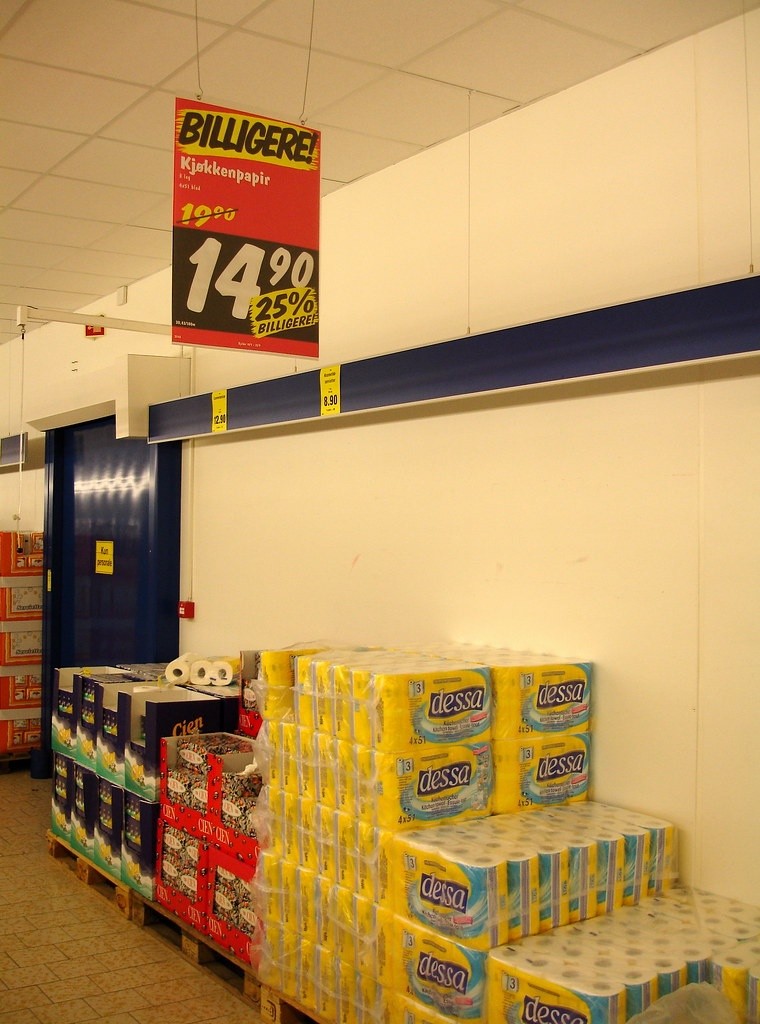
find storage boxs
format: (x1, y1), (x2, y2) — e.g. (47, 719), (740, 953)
(52, 662), (263, 970)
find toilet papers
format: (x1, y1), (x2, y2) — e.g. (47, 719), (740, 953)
(42, 636), (760, 1024)
